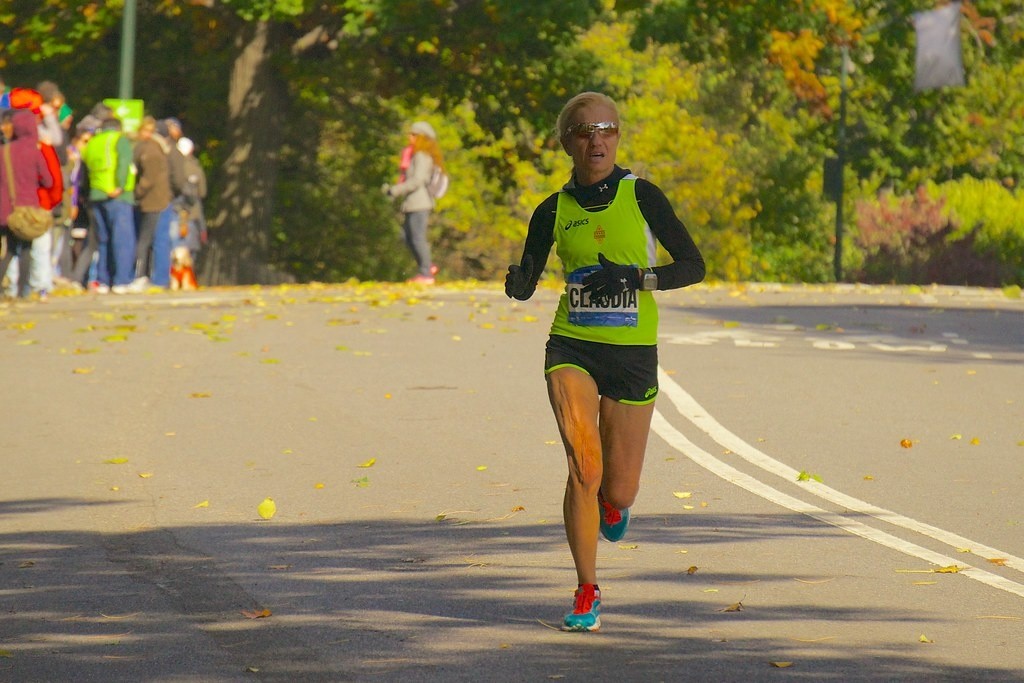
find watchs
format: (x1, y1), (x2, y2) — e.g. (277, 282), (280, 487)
(640, 267), (658, 291)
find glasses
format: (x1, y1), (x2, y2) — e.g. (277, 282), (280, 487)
(563, 122), (619, 137)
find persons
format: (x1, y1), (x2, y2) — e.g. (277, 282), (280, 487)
(382, 122), (449, 286)
(0, 77), (208, 308)
(505, 92), (707, 632)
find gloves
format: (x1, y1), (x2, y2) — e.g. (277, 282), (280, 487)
(580, 252), (641, 300)
(504, 254), (538, 301)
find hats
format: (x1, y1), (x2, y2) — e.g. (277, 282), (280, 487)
(406, 121), (437, 140)
(8, 87), (44, 110)
(176, 137), (193, 156)
(155, 119), (169, 137)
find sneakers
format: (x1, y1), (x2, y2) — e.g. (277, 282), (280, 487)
(597, 486), (630, 544)
(561, 584), (602, 632)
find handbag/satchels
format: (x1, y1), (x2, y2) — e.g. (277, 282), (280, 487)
(6, 205), (53, 241)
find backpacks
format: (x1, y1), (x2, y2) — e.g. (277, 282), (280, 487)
(425, 164), (449, 199)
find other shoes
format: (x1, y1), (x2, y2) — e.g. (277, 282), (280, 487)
(97, 286), (109, 294)
(39, 297), (48, 303)
(429, 263), (439, 276)
(111, 285), (126, 295)
(406, 273), (435, 287)
(3, 289), (18, 297)
